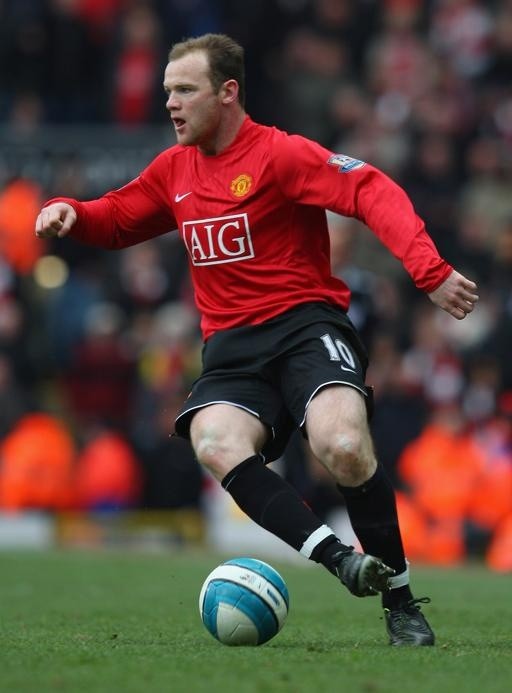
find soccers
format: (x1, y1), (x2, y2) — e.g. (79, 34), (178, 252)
(200, 558), (289, 645)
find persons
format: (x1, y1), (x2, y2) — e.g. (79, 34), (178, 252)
(35, 33), (478, 645)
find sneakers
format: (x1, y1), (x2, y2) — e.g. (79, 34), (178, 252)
(330, 546), (395, 597)
(384, 595), (435, 645)
